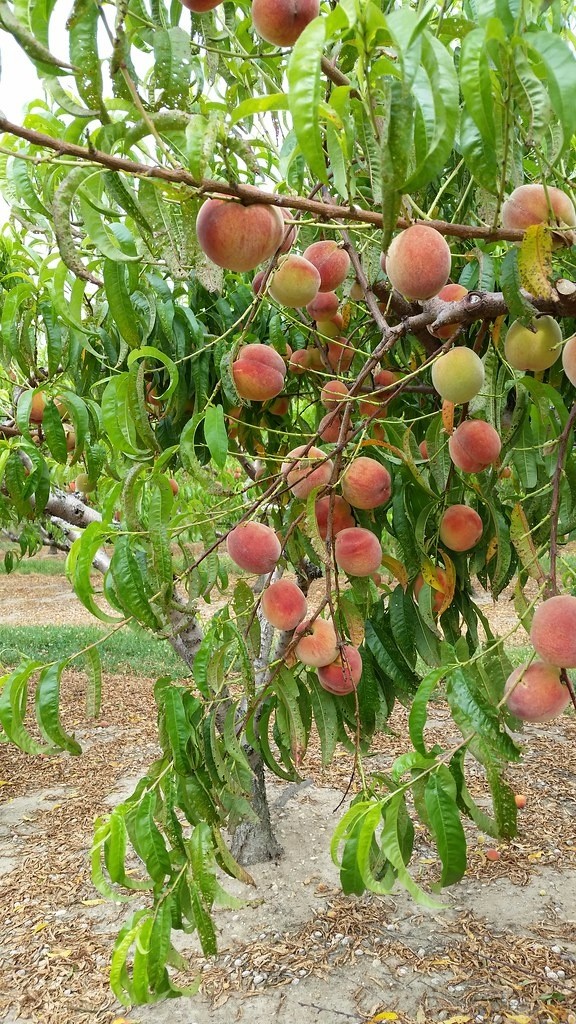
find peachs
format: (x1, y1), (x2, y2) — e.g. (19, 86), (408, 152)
(8, 1), (576, 862)
